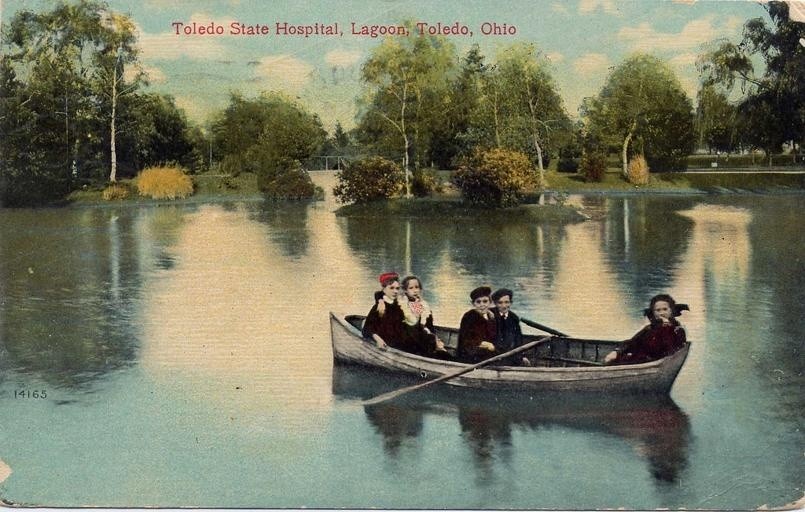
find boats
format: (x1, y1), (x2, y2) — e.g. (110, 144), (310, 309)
(329, 310), (693, 394)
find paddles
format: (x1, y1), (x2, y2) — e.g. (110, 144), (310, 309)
(359, 338), (551, 406)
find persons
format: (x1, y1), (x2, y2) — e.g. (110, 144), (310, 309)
(491, 290), (524, 365)
(362, 273), (409, 351)
(457, 288), (496, 364)
(401, 277), (452, 360)
(604, 294), (686, 368)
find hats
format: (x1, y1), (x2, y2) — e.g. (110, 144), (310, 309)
(379, 272), (399, 283)
(470, 287), (491, 299)
(492, 288), (512, 303)
